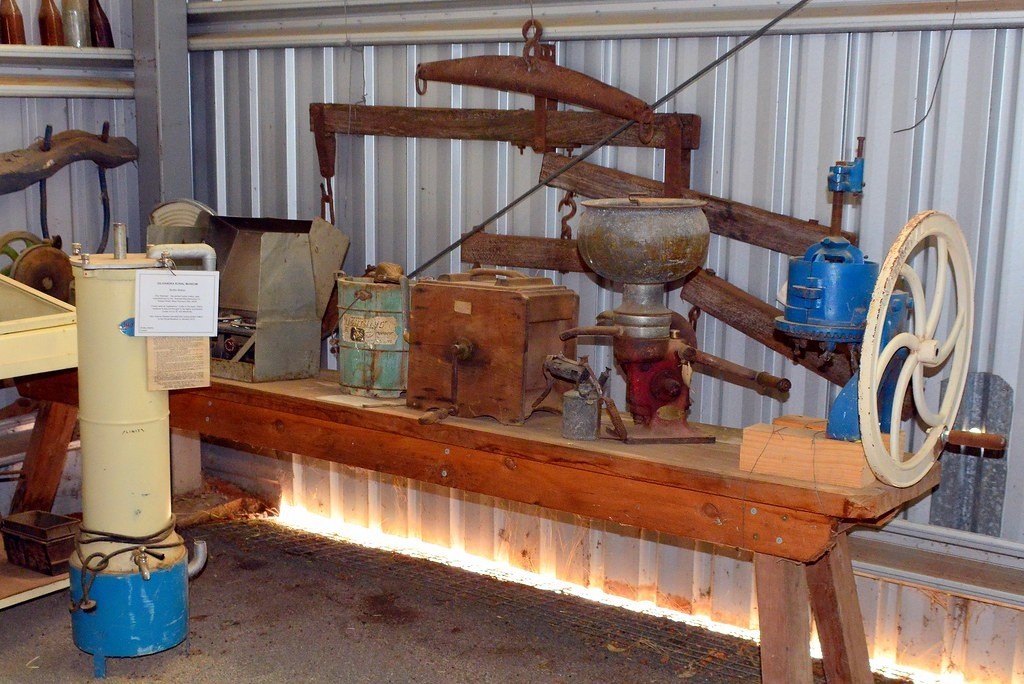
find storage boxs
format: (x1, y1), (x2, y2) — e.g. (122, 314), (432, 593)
(2, 510), (80, 575)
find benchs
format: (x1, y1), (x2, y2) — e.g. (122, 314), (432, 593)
(8, 368), (940, 684)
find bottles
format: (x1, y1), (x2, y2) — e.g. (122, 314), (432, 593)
(38, 0), (64, 46)
(88, 0), (114, 48)
(0, 0), (27, 45)
(59, 0), (92, 48)
